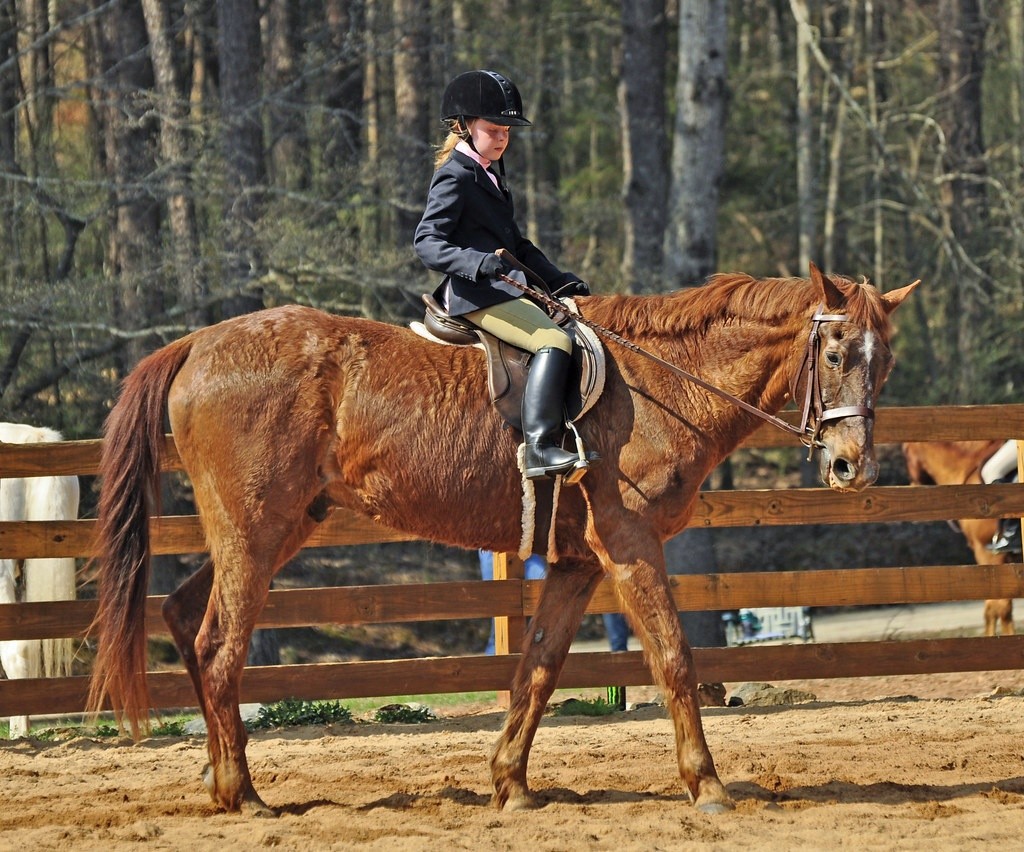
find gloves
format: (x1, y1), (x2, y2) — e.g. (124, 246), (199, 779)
(479, 253), (504, 279)
(573, 283), (591, 295)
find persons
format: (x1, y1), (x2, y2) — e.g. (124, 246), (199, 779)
(478, 549), (547, 653)
(414, 70), (605, 479)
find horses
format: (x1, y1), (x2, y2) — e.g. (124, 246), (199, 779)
(85, 261), (922, 812)
(896, 423), (1024, 642)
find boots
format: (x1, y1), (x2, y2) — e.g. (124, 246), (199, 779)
(983, 517), (1022, 554)
(521, 347), (603, 479)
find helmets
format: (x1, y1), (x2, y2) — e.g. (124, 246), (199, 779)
(441, 69), (532, 126)
